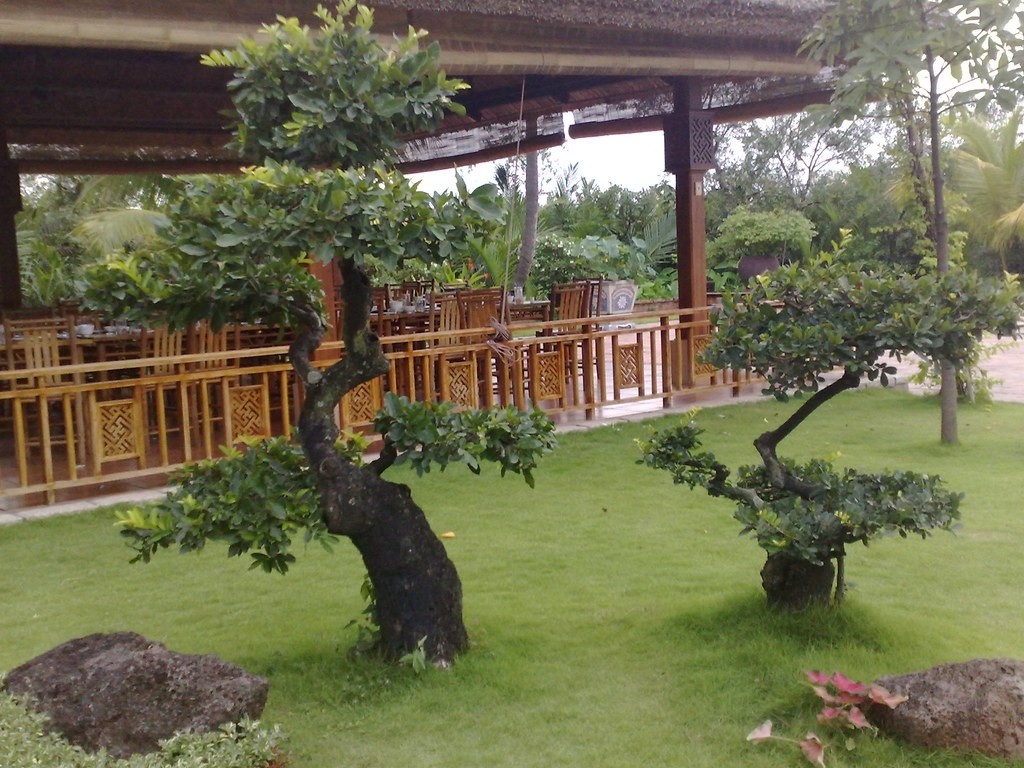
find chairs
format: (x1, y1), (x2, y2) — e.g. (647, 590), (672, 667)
(536, 276), (603, 384)
(1, 279), (536, 489)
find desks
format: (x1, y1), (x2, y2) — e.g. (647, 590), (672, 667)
(0, 298), (551, 399)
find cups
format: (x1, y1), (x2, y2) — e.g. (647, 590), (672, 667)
(80, 324), (94, 337)
(514, 286), (523, 305)
(389, 301), (403, 311)
(413, 297), (427, 312)
(507, 296), (514, 303)
(529, 296), (535, 304)
(405, 305), (416, 314)
